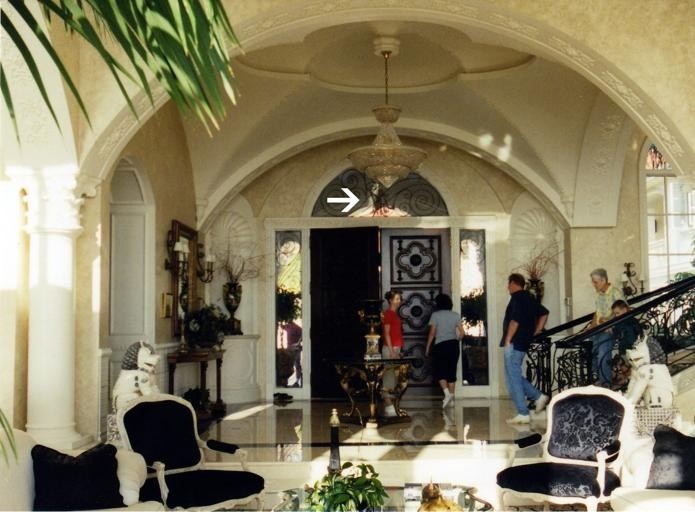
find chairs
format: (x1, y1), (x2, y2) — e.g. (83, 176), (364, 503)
(495, 385), (634, 512)
(115, 393), (266, 511)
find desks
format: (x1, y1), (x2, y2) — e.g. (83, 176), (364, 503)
(168, 349), (225, 424)
(332, 357), (411, 428)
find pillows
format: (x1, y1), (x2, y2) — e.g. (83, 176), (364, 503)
(31, 443), (128, 512)
(645, 423), (695, 491)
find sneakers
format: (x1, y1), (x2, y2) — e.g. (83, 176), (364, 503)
(532, 393), (549, 414)
(441, 393), (452, 409)
(448, 400), (455, 408)
(505, 413), (531, 426)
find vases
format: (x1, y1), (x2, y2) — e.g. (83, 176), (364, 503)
(221, 280), (243, 335)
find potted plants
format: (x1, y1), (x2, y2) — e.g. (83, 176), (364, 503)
(513, 241), (562, 303)
(181, 388), (212, 435)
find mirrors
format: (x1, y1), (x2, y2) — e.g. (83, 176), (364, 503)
(171, 218), (198, 338)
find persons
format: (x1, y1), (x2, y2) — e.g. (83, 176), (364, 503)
(281, 318), (302, 388)
(588, 268), (628, 386)
(602, 300), (642, 382)
(425, 292), (465, 408)
(381, 290), (403, 416)
(499, 272), (550, 425)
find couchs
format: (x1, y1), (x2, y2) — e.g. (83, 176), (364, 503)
(609, 424), (695, 512)
(0, 427), (165, 512)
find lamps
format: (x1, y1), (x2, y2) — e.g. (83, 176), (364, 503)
(165, 241), (190, 278)
(199, 254), (216, 283)
(620, 262), (645, 300)
(346, 49), (429, 190)
